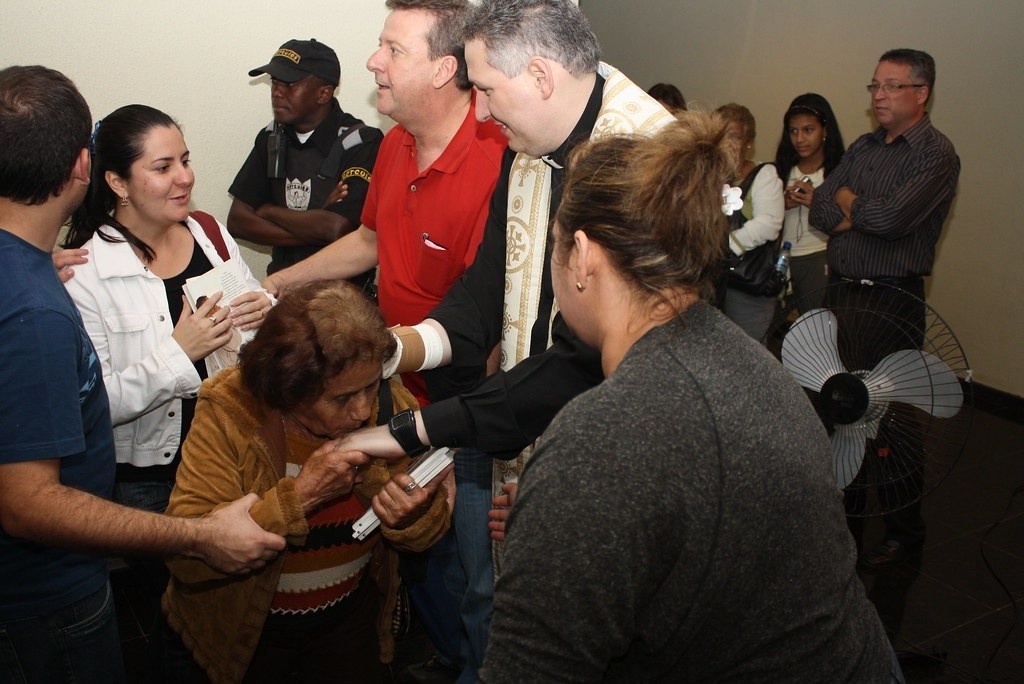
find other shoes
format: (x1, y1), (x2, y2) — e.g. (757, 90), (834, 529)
(404, 656), (462, 684)
(858, 540), (925, 566)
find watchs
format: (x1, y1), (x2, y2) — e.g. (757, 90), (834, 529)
(389, 408), (431, 457)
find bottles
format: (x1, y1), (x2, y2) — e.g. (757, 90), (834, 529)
(774, 241), (792, 276)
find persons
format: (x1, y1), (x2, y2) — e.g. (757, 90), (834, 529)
(806, 48), (962, 473)
(709, 103), (785, 354)
(334, 0), (686, 589)
(65, 104), (283, 684)
(257, 1), (508, 411)
(227, 39), (390, 293)
(161, 274), (454, 684)
(773, 93), (845, 313)
(481, 100), (904, 684)
(0, 64), (287, 684)
(647, 83), (688, 118)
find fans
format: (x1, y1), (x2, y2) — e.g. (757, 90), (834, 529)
(756, 276), (974, 584)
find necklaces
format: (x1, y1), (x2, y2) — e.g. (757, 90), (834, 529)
(280, 413), (302, 469)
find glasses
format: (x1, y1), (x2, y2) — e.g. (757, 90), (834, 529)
(867, 84), (927, 94)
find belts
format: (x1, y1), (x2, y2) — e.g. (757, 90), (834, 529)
(872, 275), (924, 288)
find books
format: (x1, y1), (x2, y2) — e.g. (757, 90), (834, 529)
(349, 446), (456, 542)
(182, 258), (261, 378)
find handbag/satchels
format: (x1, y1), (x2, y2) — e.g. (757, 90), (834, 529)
(713, 162), (789, 296)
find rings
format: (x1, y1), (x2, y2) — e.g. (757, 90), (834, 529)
(403, 481), (418, 492)
(210, 315), (216, 322)
(353, 464), (360, 470)
(258, 308), (264, 317)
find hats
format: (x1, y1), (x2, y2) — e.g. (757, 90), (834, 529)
(249, 39), (340, 85)
